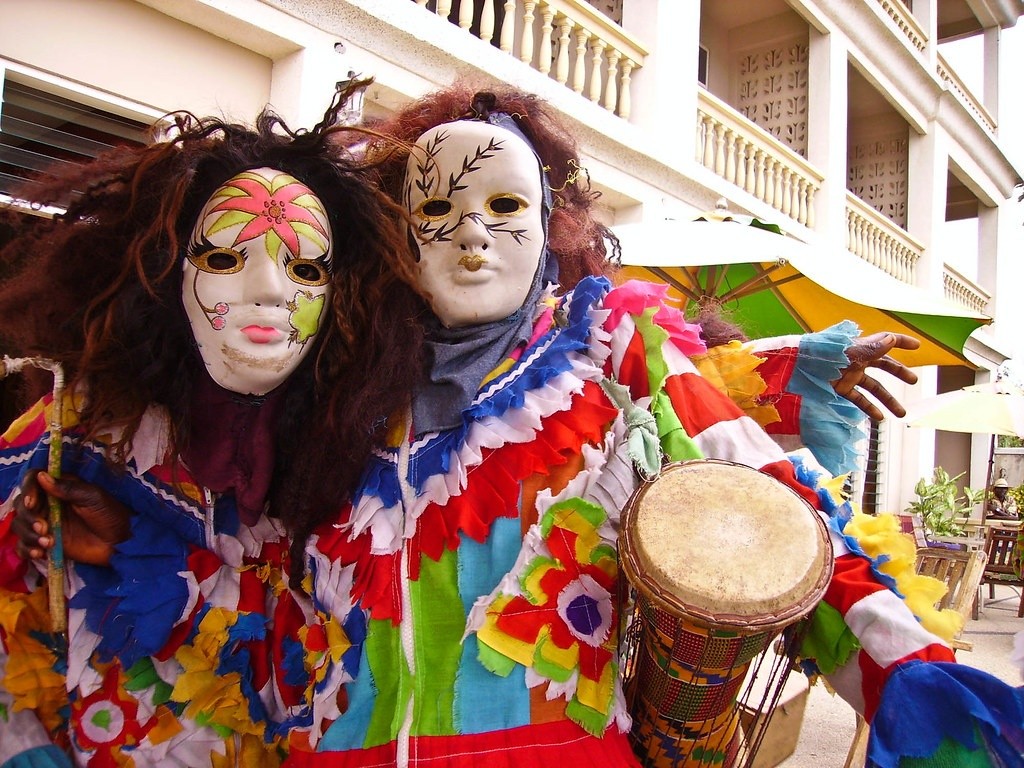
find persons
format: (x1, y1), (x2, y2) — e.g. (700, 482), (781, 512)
(0, 85), (1024, 768)
(984, 478), (1018, 553)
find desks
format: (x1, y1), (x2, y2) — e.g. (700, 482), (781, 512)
(929, 536), (1013, 613)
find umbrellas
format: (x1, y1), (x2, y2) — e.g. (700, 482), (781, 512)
(605, 198), (995, 371)
(898, 380), (1024, 539)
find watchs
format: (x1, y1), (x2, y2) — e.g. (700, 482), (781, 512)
(994, 508), (1000, 513)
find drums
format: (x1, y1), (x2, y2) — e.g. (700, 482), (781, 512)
(617, 455), (836, 768)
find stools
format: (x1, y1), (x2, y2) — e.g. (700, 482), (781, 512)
(737, 660), (811, 768)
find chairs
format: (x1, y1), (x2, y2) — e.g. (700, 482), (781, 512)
(844, 515), (1024, 768)
